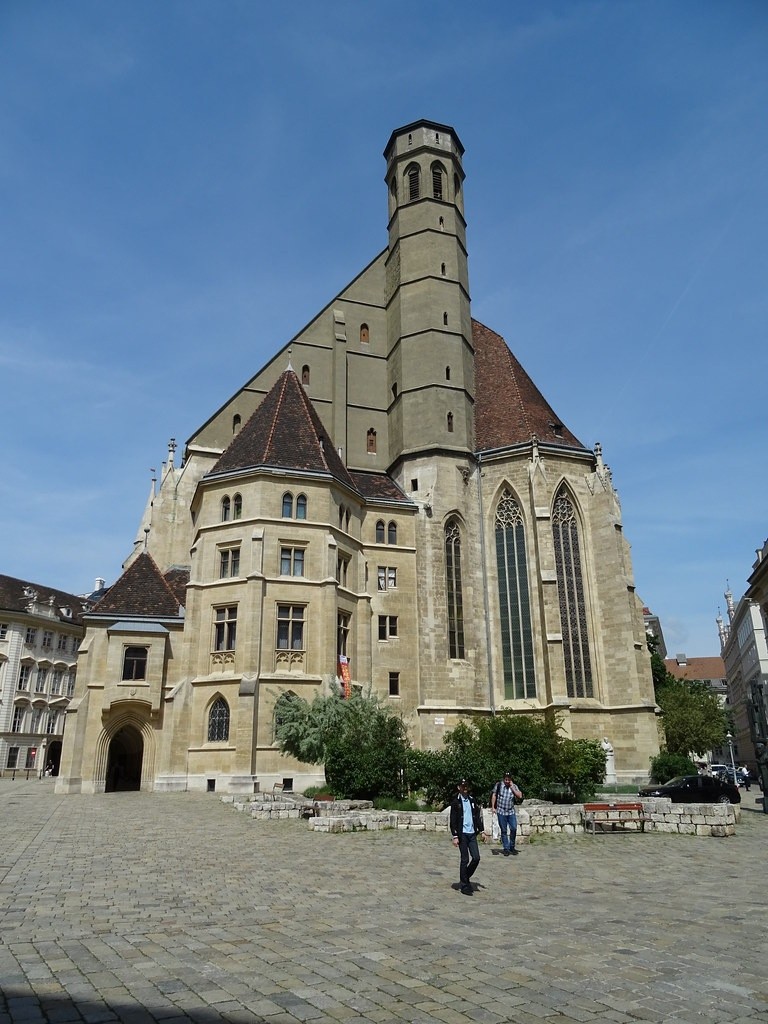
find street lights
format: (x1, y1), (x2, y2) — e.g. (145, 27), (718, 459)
(727, 730), (741, 787)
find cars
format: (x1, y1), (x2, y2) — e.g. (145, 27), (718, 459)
(698, 762), (747, 787)
(637, 774), (742, 805)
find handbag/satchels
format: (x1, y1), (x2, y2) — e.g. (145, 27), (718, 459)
(494, 782), (501, 809)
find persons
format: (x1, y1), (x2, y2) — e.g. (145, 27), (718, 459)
(491, 772), (523, 856)
(742, 763), (751, 792)
(45, 759), (54, 778)
(450, 778), (486, 896)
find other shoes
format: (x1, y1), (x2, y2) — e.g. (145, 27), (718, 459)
(504, 849), (509, 856)
(461, 882), (473, 896)
(510, 848), (517, 855)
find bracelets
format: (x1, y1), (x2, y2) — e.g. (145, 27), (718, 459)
(452, 836), (458, 839)
(480, 831), (485, 835)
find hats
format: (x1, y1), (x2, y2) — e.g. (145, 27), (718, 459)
(503, 770), (511, 777)
(458, 778), (468, 786)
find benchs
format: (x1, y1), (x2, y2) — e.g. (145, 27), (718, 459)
(301, 794), (336, 818)
(263, 783), (285, 802)
(584, 802), (652, 836)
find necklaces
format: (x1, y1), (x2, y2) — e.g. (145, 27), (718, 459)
(464, 800), (468, 802)
